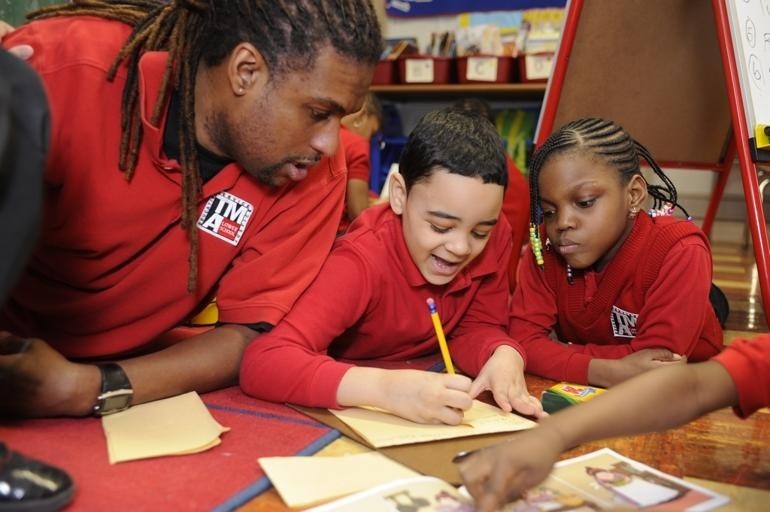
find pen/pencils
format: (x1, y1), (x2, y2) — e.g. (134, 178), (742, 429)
(429, 32), (457, 60)
(426, 298), (455, 376)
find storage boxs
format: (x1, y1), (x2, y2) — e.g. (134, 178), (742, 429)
(370, 59), (398, 85)
(397, 55), (457, 84)
(456, 55), (518, 83)
(518, 51), (555, 83)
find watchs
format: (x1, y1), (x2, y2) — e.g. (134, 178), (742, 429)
(89, 361), (134, 418)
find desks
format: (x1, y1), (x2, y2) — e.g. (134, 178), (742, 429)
(0, 372), (770, 512)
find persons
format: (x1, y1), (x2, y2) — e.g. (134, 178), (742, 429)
(336, 90), (383, 237)
(0, 0), (383, 421)
(238, 108), (550, 426)
(454, 330), (768, 512)
(506, 115), (730, 389)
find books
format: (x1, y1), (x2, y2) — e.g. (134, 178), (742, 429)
(304, 446), (730, 511)
